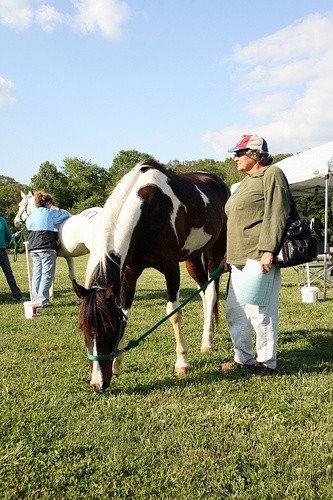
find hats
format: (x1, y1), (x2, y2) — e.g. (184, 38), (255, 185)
(227, 134), (269, 154)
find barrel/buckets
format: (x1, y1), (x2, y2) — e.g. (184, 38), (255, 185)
(24, 301), (42, 319)
(301, 287), (318, 303)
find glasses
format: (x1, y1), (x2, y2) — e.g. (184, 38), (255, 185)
(233, 151), (249, 158)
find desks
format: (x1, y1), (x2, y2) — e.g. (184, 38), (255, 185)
(296, 263), (333, 288)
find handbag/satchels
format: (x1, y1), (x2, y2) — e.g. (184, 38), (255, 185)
(273, 216), (323, 269)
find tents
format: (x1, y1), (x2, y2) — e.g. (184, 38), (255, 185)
(231, 139), (333, 301)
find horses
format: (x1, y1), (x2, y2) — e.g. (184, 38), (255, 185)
(12, 189), (105, 288)
(71, 156), (231, 395)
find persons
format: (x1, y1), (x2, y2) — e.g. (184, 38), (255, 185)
(0, 204), (28, 300)
(28, 192), (70, 308)
(221, 134), (291, 375)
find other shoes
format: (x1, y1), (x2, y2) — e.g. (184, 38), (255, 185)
(13, 296), (30, 301)
(246, 364), (276, 380)
(218, 359), (255, 372)
